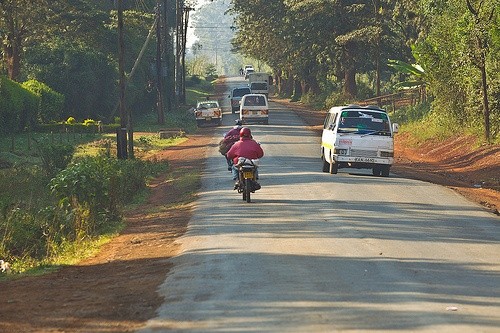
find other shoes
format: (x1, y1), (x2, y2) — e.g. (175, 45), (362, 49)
(233, 180), (239, 189)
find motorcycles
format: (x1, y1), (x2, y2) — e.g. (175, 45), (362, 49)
(225, 143), (260, 203)
(222, 132), (238, 174)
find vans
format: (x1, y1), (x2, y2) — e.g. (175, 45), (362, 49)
(228, 87), (252, 114)
(245, 68), (255, 80)
(320, 103), (394, 177)
(239, 94), (269, 125)
(243, 65), (254, 75)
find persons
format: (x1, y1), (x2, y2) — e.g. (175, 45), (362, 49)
(338, 117), (350, 128)
(227, 128), (264, 190)
(240, 68), (243, 75)
(223, 119), (252, 171)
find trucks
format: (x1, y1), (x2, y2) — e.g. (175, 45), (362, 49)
(248, 72), (273, 102)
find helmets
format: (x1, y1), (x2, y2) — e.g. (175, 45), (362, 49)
(234, 118), (242, 125)
(239, 127), (251, 138)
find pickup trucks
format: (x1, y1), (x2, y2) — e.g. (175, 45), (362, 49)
(194, 101), (223, 128)
